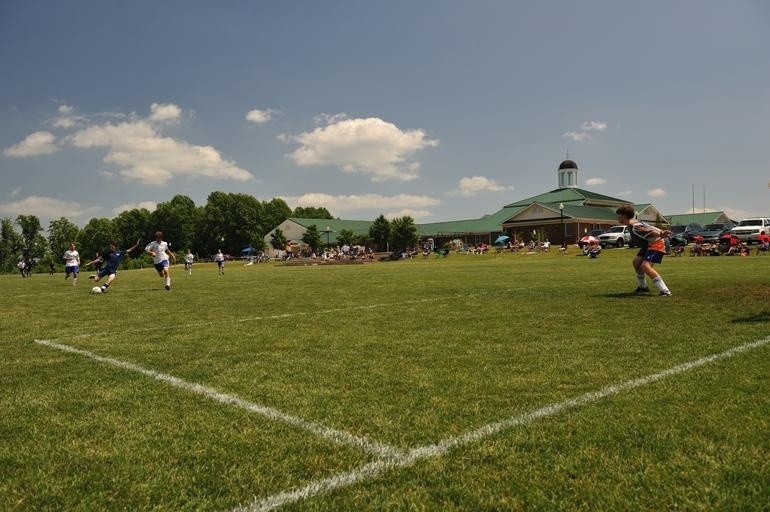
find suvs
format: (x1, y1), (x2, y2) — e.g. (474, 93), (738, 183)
(596, 224), (632, 247)
(731, 216), (770, 244)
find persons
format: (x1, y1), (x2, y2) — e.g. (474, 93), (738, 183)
(665, 231), (769, 256)
(470, 243), (488, 255)
(250, 249), (311, 263)
(18, 259), (32, 278)
(63, 243), (80, 286)
(311, 243), (375, 263)
(184, 249), (194, 276)
(49, 260), (55, 275)
(615, 206), (672, 297)
(145, 232), (176, 290)
(402, 244), (430, 259)
(215, 250), (224, 275)
(85, 238), (140, 292)
(583, 242), (602, 258)
(499, 239), (567, 253)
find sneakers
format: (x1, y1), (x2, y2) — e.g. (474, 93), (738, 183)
(165, 286), (170, 290)
(635, 286), (672, 296)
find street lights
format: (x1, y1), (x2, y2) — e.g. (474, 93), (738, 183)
(327, 226), (330, 251)
(558, 203), (565, 247)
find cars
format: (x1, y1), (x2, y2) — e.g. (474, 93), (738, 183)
(694, 222), (732, 245)
(578, 230), (604, 249)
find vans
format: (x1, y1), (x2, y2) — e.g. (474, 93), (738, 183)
(667, 223), (702, 246)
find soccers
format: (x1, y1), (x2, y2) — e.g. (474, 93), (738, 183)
(92, 287), (102, 293)
